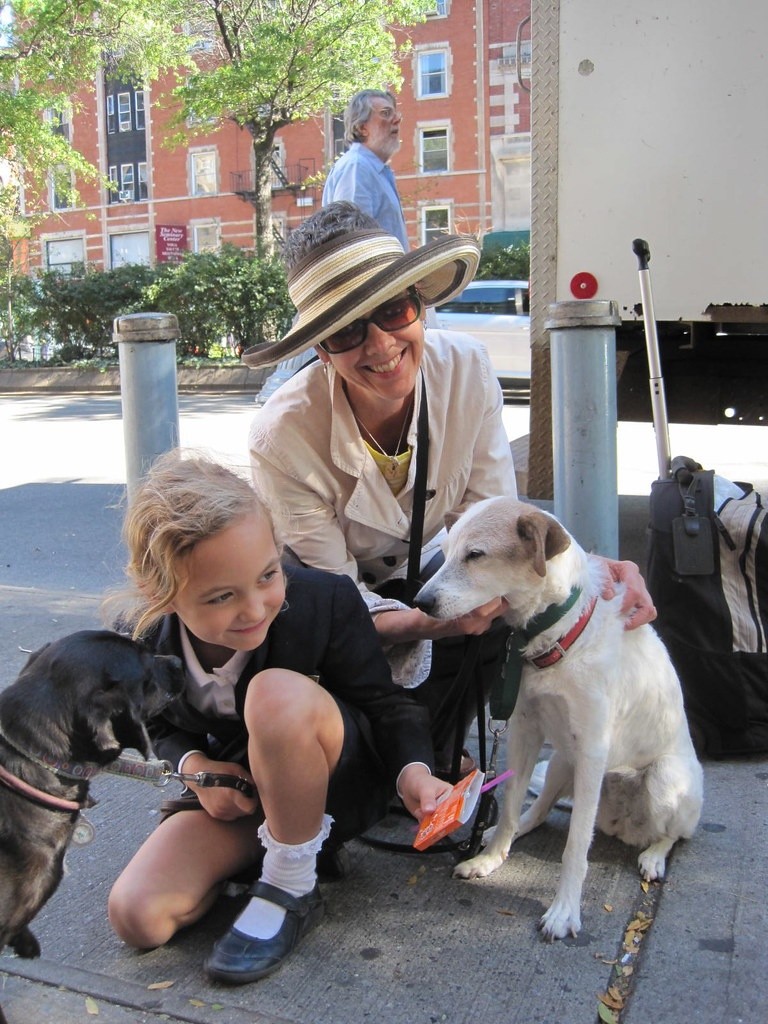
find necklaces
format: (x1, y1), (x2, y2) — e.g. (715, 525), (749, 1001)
(355, 395), (413, 471)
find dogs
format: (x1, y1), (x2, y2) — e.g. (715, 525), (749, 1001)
(413, 495), (706, 943)
(0, 629), (185, 961)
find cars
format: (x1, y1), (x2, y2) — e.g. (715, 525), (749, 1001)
(424, 281), (531, 400)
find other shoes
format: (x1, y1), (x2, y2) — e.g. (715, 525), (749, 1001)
(435, 749), (476, 778)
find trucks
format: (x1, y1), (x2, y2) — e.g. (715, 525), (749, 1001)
(516, 1), (768, 499)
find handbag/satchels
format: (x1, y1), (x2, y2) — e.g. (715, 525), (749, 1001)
(369, 578), (422, 608)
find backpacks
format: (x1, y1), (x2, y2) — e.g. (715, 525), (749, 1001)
(644, 455), (768, 758)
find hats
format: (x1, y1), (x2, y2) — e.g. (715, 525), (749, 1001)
(241, 228), (482, 368)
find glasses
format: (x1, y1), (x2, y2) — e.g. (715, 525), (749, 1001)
(319, 287), (421, 354)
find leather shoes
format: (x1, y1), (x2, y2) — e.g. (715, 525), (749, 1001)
(203, 882), (325, 982)
(315, 834), (351, 881)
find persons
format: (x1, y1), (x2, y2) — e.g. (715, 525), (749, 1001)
(107, 453), (455, 985)
(244, 201), (657, 780)
(320, 90), (411, 254)
